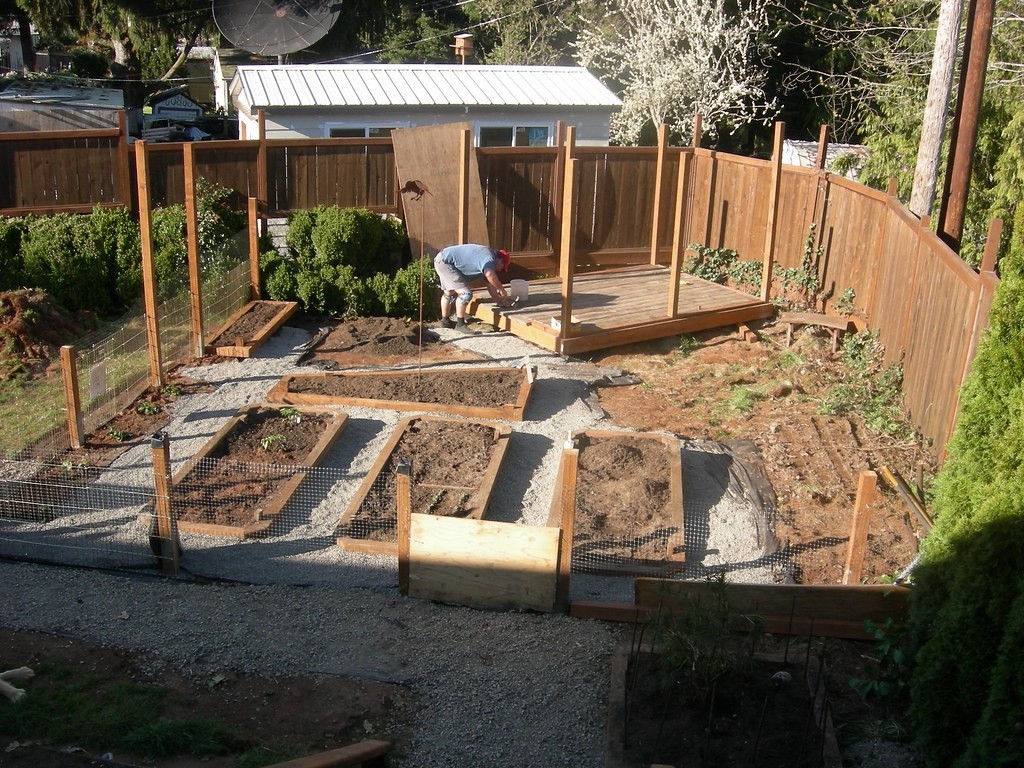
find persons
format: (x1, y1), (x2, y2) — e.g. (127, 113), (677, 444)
(434, 245), (510, 334)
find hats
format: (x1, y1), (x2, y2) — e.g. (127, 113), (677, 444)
(494, 249), (510, 272)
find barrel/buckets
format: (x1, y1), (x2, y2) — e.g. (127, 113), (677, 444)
(510, 278), (529, 302)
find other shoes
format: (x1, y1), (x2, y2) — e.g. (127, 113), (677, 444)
(441, 321), (457, 328)
(455, 324), (474, 335)
